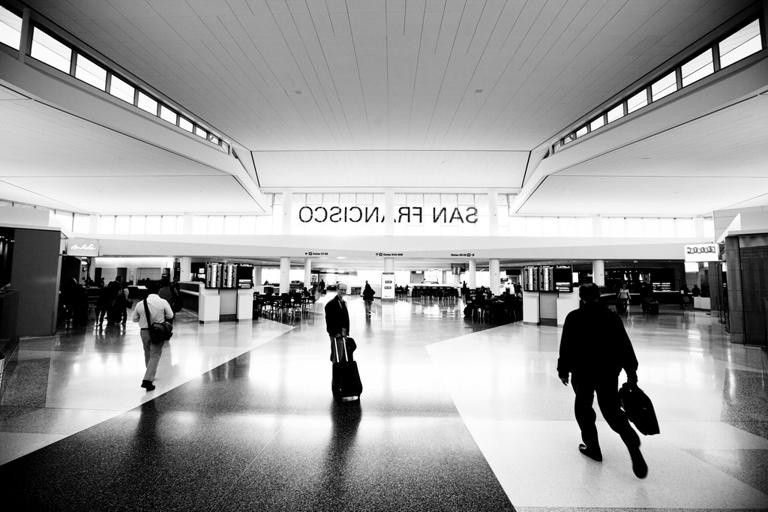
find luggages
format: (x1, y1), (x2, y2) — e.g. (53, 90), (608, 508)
(333, 361), (362, 398)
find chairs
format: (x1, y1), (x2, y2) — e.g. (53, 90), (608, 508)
(461, 285), (523, 329)
(411, 286), (460, 304)
(257, 288), (316, 323)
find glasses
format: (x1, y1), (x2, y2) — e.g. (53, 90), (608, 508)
(337, 287), (348, 292)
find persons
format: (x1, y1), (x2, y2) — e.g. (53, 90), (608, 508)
(132, 283), (173, 389)
(640, 281), (654, 315)
(362, 281), (376, 306)
(325, 283), (349, 362)
(299, 279), (326, 303)
(557, 282), (647, 478)
(68, 274), (183, 332)
(692, 284), (700, 296)
(615, 282), (631, 314)
(679, 284), (691, 310)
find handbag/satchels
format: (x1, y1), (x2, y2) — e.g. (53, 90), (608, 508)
(147, 319), (174, 342)
(620, 386), (663, 438)
(330, 337), (357, 361)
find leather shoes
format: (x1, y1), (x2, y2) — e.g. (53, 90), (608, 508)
(577, 442), (603, 463)
(626, 450), (649, 481)
(139, 381), (158, 391)
